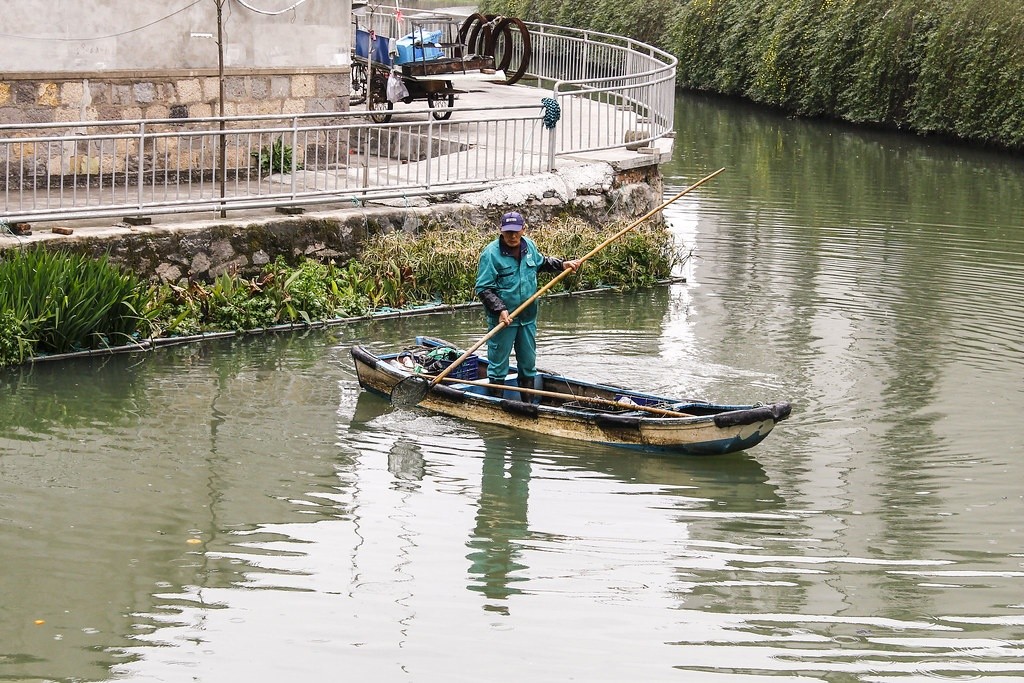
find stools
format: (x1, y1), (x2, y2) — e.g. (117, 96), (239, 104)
(394, 29), (445, 66)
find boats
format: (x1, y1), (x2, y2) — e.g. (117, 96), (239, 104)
(351, 333), (792, 458)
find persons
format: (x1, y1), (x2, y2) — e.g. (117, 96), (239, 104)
(474, 212), (580, 403)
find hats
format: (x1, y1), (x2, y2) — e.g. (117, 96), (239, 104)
(500, 211), (524, 232)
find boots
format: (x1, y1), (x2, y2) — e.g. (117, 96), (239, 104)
(516, 374), (534, 403)
(490, 378), (504, 398)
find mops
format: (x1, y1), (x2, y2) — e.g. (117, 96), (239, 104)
(510, 97), (561, 177)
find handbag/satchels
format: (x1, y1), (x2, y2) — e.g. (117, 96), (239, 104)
(386, 69), (409, 102)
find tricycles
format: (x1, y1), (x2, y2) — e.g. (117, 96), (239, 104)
(349, 38), (457, 124)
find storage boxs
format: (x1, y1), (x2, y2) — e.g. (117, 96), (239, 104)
(437, 355), (479, 384)
